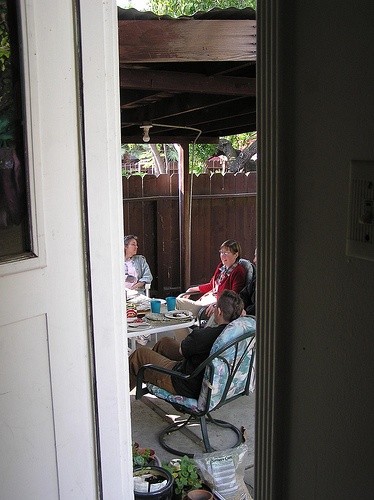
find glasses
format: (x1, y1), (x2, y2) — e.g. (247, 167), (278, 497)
(219, 251), (235, 255)
(213, 304), (218, 308)
(128, 244), (139, 246)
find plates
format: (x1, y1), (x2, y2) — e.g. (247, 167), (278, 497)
(164, 309), (193, 319)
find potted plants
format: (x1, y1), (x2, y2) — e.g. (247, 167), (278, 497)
(161, 456), (203, 500)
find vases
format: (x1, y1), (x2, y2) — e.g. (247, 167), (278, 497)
(133, 466), (175, 500)
(133, 455), (162, 469)
(187, 489), (214, 500)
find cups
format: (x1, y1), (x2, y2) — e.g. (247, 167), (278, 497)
(150, 301), (161, 314)
(166, 297), (177, 311)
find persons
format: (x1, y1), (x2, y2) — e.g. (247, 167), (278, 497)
(253, 248), (257, 265)
(124, 234), (153, 311)
(128, 288), (244, 398)
(175, 239), (248, 318)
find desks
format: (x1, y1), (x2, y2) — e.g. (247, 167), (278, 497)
(127, 305), (195, 354)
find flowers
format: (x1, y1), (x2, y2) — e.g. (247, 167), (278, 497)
(132, 441), (156, 467)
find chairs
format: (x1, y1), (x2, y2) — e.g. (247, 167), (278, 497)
(176, 259), (257, 319)
(136, 315), (255, 458)
